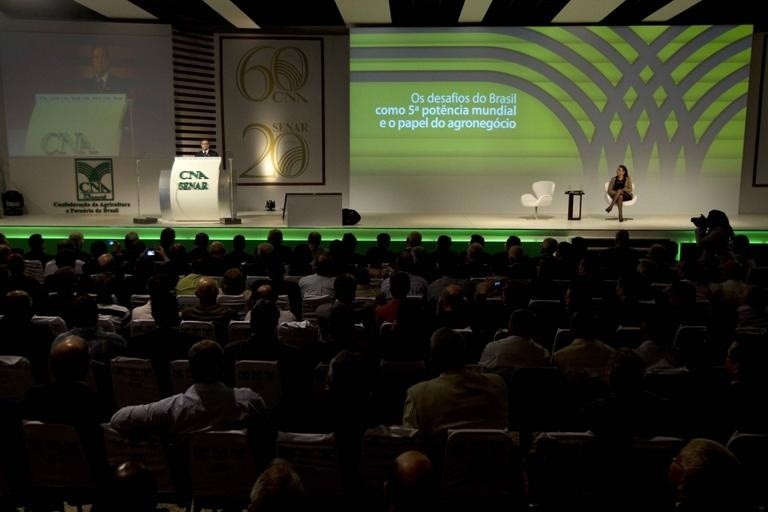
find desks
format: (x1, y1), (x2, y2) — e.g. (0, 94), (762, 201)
(564, 189), (585, 219)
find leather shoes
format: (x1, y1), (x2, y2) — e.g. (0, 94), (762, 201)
(605, 208), (610, 213)
(620, 218), (623, 221)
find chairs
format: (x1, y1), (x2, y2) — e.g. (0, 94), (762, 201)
(605, 180), (636, 219)
(521, 180), (556, 219)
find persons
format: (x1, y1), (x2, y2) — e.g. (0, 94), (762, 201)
(79, 45), (124, 93)
(694, 209), (738, 264)
(1, 226), (768, 509)
(606, 164), (633, 224)
(193, 139), (219, 157)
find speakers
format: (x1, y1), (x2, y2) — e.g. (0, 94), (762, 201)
(342, 208), (362, 226)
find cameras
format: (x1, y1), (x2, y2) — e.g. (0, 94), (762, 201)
(493, 280), (502, 289)
(692, 214), (708, 227)
(145, 249), (158, 257)
(107, 240), (115, 247)
